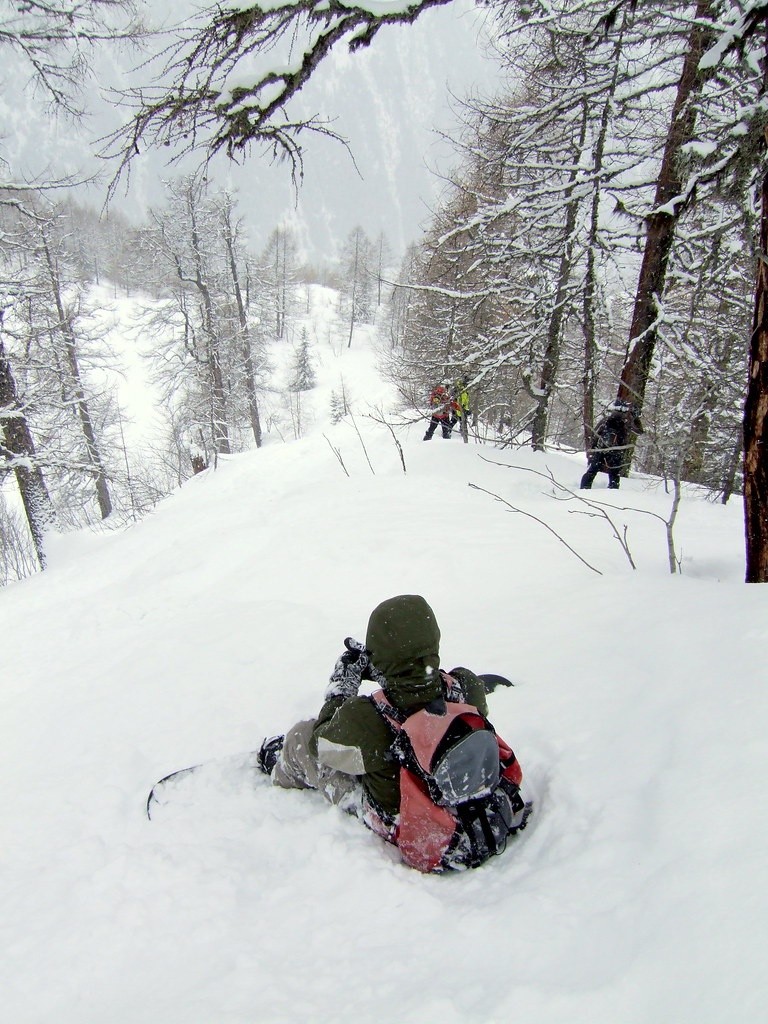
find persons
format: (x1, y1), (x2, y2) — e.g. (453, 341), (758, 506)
(422, 377), (471, 440)
(580, 401), (644, 490)
(258, 595), (488, 845)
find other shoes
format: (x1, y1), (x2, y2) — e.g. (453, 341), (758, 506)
(260, 734), (285, 773)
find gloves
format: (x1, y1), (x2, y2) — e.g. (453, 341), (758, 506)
(324, 650), (370, 697)
(630, 405), (642, 417)
(343, 637), (386, 685)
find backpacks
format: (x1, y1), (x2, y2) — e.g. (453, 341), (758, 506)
(595, 417), (627, 455)
(367, 671), (525, 872)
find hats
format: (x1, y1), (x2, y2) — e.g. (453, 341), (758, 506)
(614, 401), (629, 414)
(442, 379), (451, 385)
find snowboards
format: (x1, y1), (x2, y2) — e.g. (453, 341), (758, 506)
(146, 749), (259, 820)
